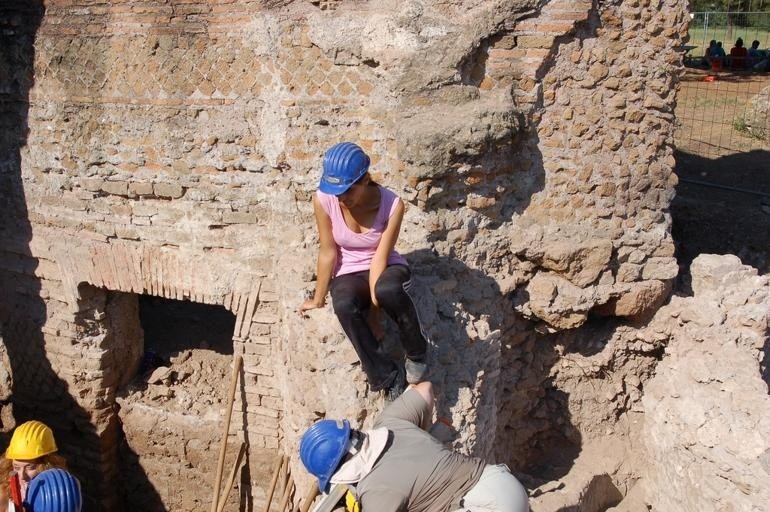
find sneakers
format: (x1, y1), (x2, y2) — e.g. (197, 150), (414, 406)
(405, 359), (426, 384)
(384, 368), (405, 400)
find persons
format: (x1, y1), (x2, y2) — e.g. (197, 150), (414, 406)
(700, 37), (770, 72)
(299, 378), (533, 511)
(4, 419), (59, 511)
(298, 140), (428, 403)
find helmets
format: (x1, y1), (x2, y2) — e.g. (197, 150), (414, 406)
(320, 142), (370, 195)
(299, 419), (350, 492)
(6, 420), (57, 460)
(26, 468), (82, 512)
